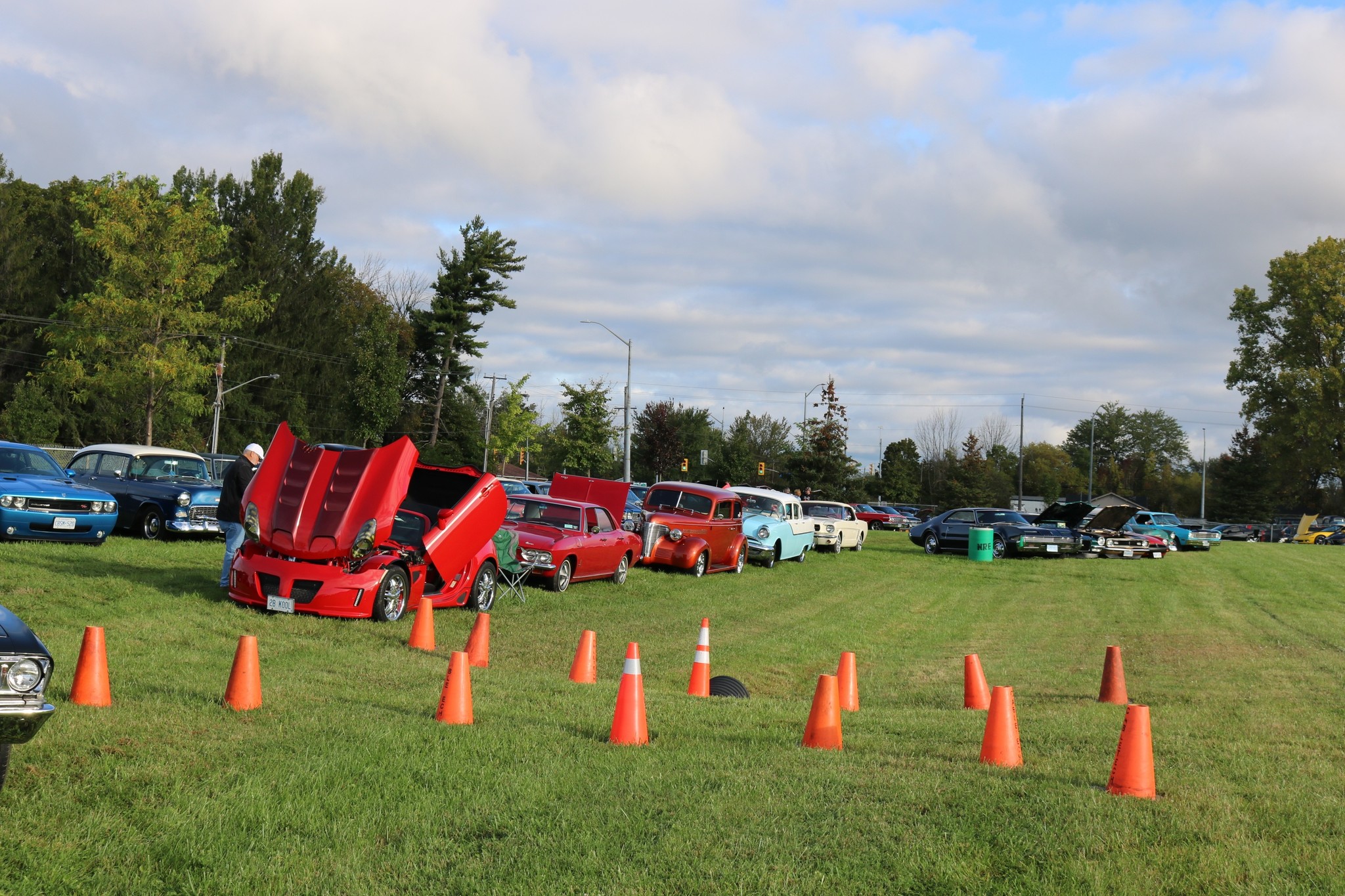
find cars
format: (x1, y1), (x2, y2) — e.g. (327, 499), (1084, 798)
(1211, 513), (1345, 546)
(0, 605), (56, 791)
(63, 443), (261, 539)
(843, 504), (934, 531)
(909, 500), (1221, 559)
(0, 440), (118, 546)
(492, 472), (651, 592)
(635, 481), (868, 580)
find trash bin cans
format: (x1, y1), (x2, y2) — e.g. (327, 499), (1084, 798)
(968, 527), (994, 562)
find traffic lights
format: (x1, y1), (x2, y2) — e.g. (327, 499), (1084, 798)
(520, 447), (524, 465)
(758, 462), (765, 476)
(681, 459), (688, 471)
(869, 464), (875, 476)
(493, 446), (498, 461)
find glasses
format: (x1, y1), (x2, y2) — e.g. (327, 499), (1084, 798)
(258, 455), (261, 461)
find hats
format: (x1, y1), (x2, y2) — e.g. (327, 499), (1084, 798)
(805, 487), (811, 491)
(245, 443), (264, 460)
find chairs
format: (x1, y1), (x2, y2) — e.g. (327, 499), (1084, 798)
(492, 530), (534, 603)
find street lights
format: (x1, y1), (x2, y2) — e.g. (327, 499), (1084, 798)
(1200, 428), (1206, 519)
(803, 384), (826, 445)
(844, 418), (852, 457)
(212, 374), (280, 454)
(580, 321), (632, 483)
(1088, 404), (1106, 505)
(690, 406), (725, 442)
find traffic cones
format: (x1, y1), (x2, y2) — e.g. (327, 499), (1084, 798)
(964, 653), (1155, 804)
(409, 597), (710, 745)
(801, 651), (860, 750)
(69, 626), (262, 707)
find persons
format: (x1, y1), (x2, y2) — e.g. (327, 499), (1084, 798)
(783, 486), (815, 501)
(216, 442), (264, 591)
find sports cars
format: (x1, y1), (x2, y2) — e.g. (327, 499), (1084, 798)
(228, 421), (508, 622)
